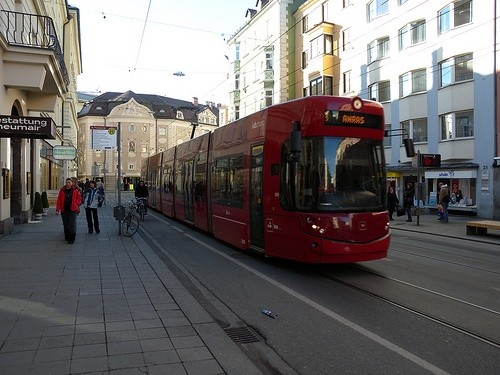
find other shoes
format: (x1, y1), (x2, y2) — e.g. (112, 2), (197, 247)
(389, 217), (394, 221)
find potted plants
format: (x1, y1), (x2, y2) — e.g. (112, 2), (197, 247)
(34, 192), (43, 220)
(41, 192), (49, 215)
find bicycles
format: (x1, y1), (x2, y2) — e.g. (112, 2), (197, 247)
(136, 196), (148, 221)
(122, 197), (143, 237)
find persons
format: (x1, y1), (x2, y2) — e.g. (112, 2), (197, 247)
(450, 188), (458, 204)
(81, 181), (104, 233)
(456, 190), (463, 203)
(439, 180), (450, 224)
(437, 183), (444, 220)
(388, 186), (400, 221)
(135, 181), (149, 215)
(69, 178), (105, 208)
(55, 178), (81, 245)
(404, 183), (414, 222)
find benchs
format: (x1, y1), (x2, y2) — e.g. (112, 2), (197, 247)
(466, 220), (500, 237)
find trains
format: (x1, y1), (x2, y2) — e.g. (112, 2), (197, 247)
(142, 94), (415, 267)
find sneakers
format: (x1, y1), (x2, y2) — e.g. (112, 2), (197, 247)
(406, 218), (412, 222)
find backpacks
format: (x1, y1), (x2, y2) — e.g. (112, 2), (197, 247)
(442, 193), (451, 204)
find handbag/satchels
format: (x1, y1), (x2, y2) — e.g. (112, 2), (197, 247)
(396, 204), (405, 216)
